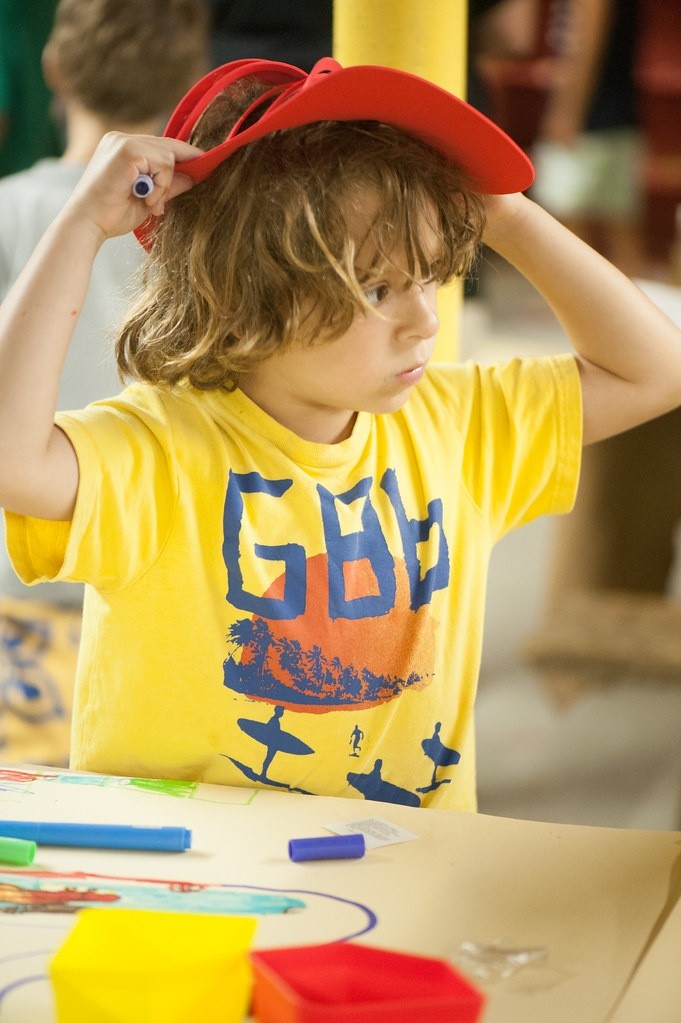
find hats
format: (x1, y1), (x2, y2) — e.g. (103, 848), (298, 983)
(131, 56), (536, 256)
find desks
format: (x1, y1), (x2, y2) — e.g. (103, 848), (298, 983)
(0, 761), (681, 1023)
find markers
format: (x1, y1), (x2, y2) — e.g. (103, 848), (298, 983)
(0, 820), (192, 867)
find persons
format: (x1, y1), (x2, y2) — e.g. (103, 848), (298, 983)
(0, 1), (208, 608)
(1, 56), (681, 813)
